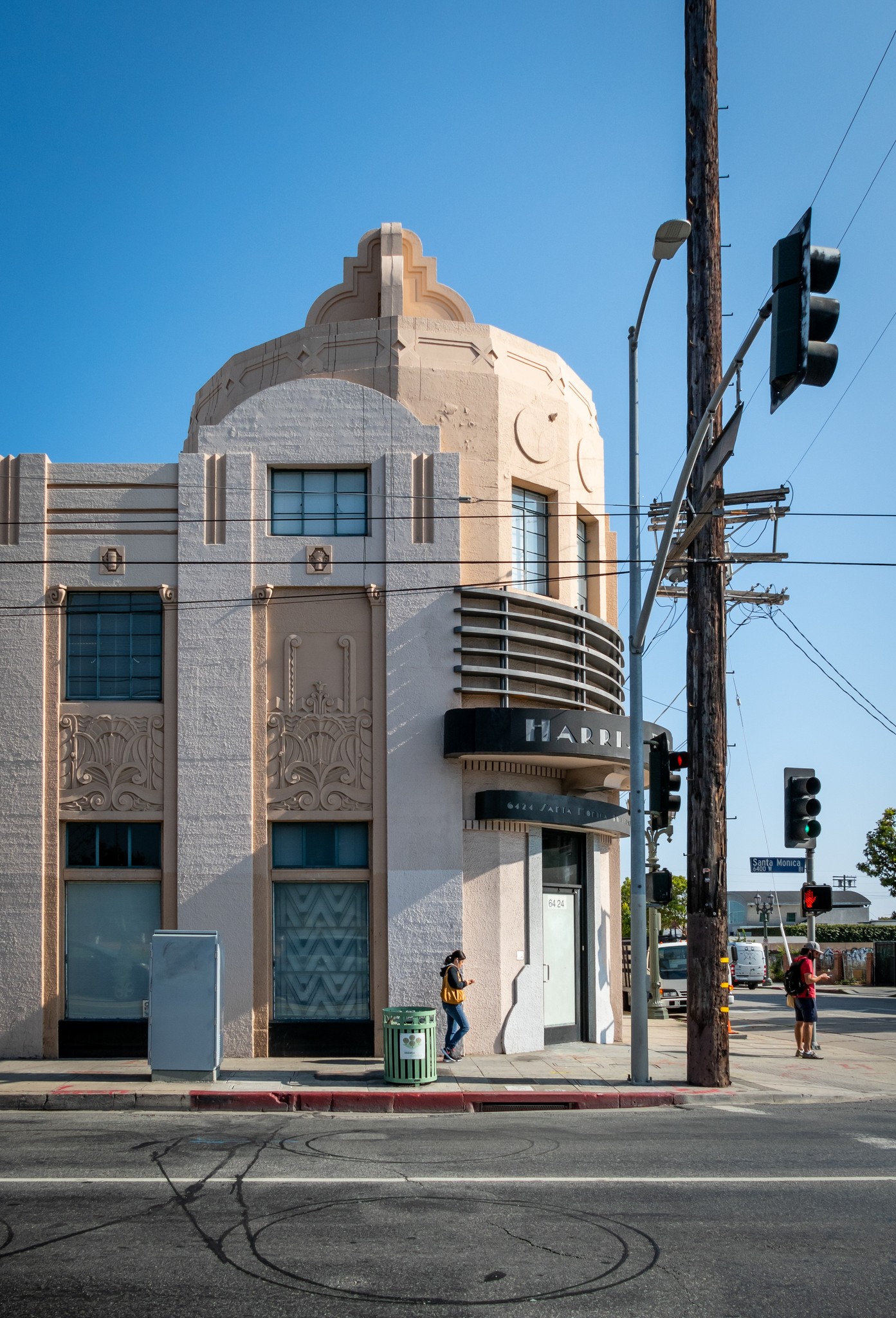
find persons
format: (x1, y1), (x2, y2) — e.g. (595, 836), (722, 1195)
(440, 950), (475, 1062)
(791, 941), (831, 1059)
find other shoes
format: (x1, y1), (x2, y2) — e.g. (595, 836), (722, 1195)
(795, 1050), (803, 1057)
(802, 1051), (824, 1059)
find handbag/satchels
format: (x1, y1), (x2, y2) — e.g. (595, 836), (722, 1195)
(441, 965), (465, 1004)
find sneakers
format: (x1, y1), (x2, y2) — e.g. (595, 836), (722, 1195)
(443, 1054), (462, 1062)
(442, 1046), (457, 1061)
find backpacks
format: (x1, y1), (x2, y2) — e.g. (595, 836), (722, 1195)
(783, 957), (809, 995)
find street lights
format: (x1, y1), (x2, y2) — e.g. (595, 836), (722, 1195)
(753, 891), (775, 987)
(627, 220), (693, 1088)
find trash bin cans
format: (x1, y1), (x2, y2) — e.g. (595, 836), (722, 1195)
(383, 1006), (439, 1085)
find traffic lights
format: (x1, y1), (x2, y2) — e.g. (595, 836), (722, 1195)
(767, 207), (842, 415)
(651, 870), (672, 903)
(647, 733), (688, 830)
(783, 767), (821, 848)
(799, 884), (835, 912)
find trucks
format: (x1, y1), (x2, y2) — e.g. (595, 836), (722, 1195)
(621, 933), (733, 1013)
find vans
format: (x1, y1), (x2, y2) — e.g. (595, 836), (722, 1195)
(725, 942), (766, 990)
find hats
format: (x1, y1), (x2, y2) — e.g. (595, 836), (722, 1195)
(804, 941), (824, 954)
